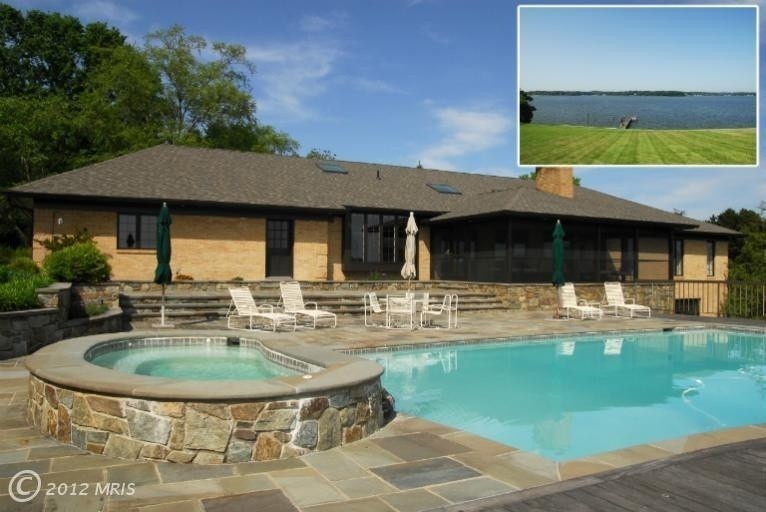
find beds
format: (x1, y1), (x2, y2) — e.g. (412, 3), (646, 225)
(580, 301), (600, 317)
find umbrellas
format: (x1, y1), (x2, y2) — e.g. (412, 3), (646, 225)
(401, 211), (419, 300)
(549, 219), (566, 318)
(154, 200), (174, 325)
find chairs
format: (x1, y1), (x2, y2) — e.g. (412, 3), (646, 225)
(364, 290), (458, 329)
(603, 336), (624, 354)
(361, 347), (459, 376)
(558, 281), (602, 320)
(225, 287), (296, 332)
(278, 282), (337, 329)
(601, 280), (652, 319)
(556, 342), (574, 355)
